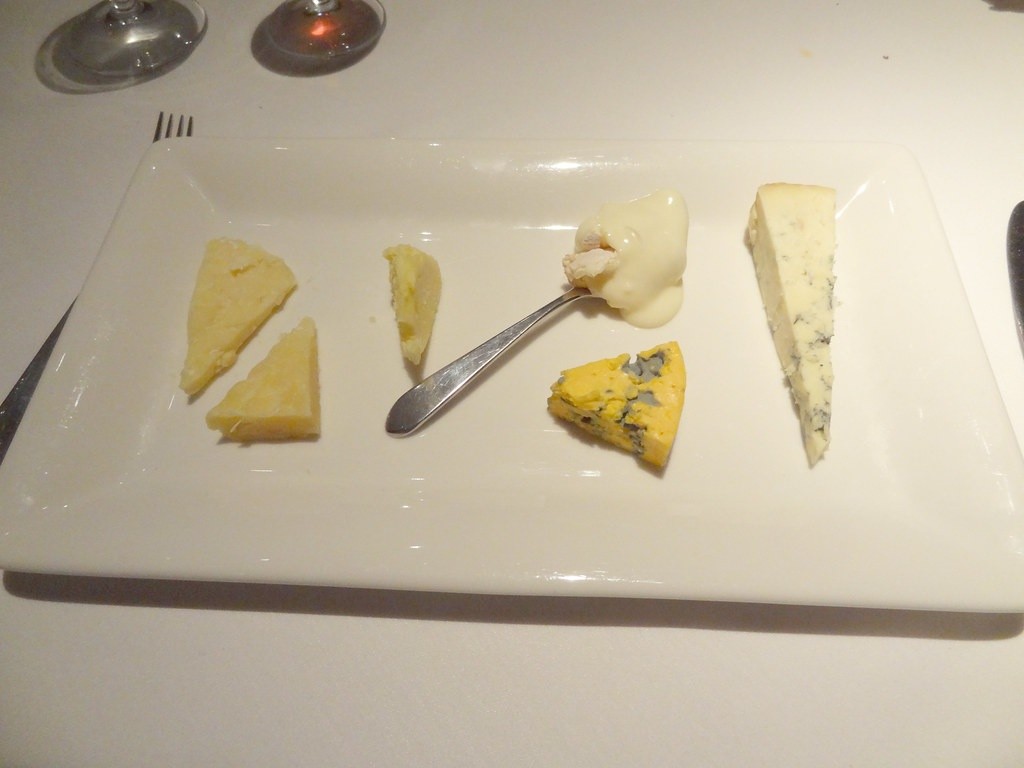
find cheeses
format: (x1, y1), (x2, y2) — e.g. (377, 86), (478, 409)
(180, 180), (836, 467)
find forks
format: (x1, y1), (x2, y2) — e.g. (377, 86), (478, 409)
(0, 110), (195, 464)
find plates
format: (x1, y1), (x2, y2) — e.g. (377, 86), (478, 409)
(0, 137), (1023, 614)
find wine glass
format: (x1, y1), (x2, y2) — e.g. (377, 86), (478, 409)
(268, 0), (387, 61)
(66, 0), (208, 80)
